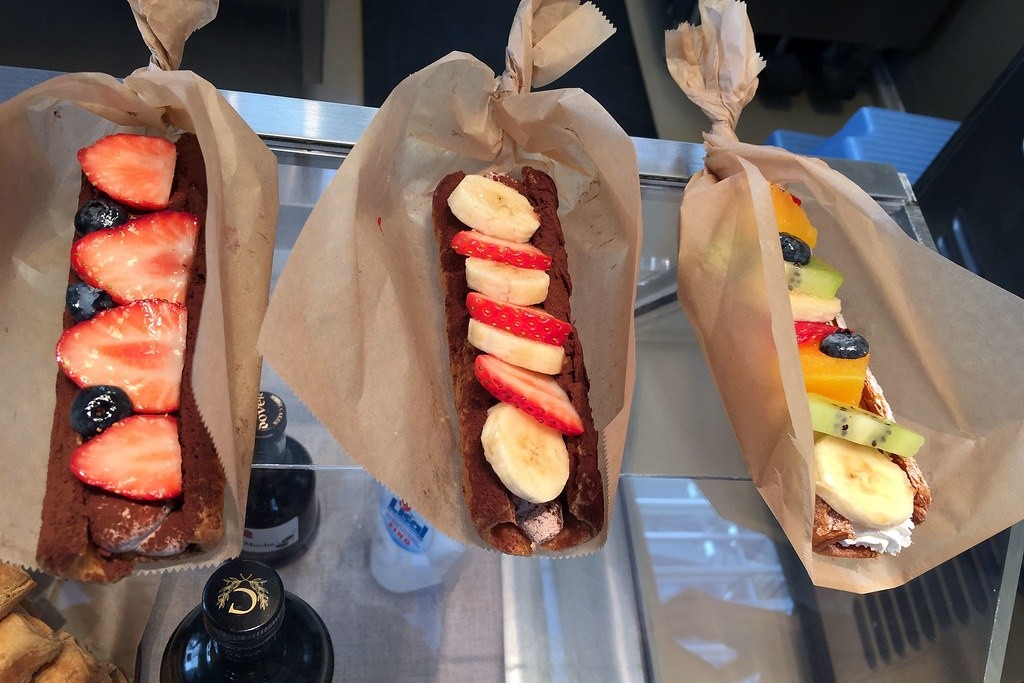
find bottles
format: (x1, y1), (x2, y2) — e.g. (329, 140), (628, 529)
(237, 391), (321, 570)
(369, 480), (468, 593)
(159, 559), (335, 683)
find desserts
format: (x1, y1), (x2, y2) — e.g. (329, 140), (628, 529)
(431, 165), (607, 556)
(767, 177), (931, 556)
(32, 132), (226, 577)
